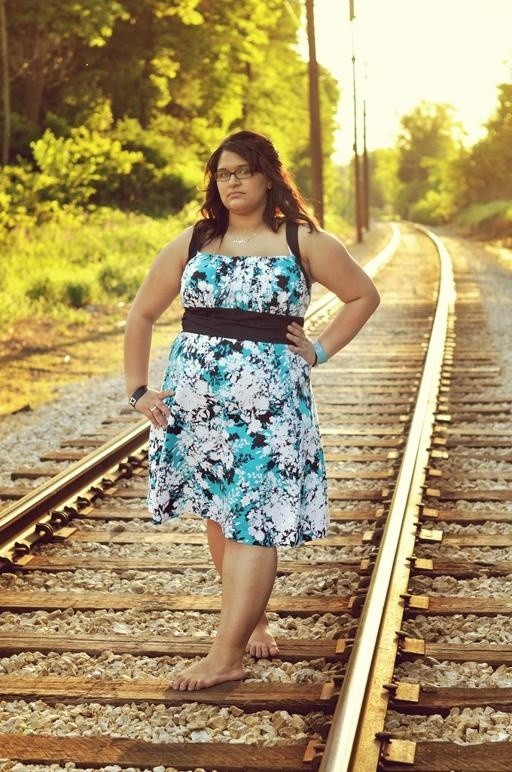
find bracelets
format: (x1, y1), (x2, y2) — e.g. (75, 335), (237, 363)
(129, 385), (149, 408)
(313, 338), (328, 364)
(312, 352), (317, 368)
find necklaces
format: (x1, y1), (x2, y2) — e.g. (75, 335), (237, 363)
(226, 225), (267, 244)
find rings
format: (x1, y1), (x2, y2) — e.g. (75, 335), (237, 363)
(151, 407), (156, 412)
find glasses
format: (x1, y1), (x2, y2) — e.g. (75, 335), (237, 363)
(211, 165), (269, 184)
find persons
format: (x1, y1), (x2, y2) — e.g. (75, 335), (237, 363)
(125, 131), (380, 691)
